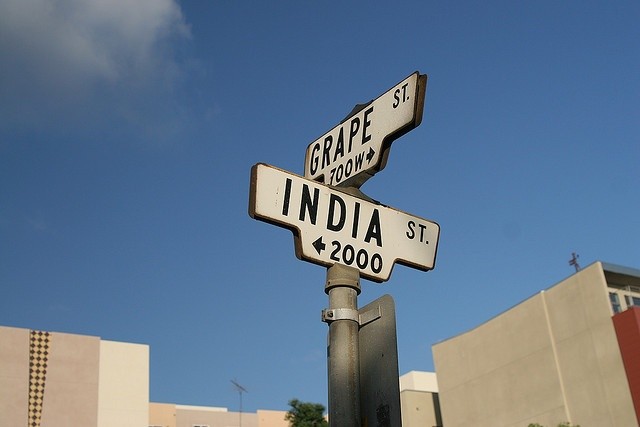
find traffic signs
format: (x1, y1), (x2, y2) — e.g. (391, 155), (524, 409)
(303, 70), (427, 189)
(246, 159), (441, 285)
